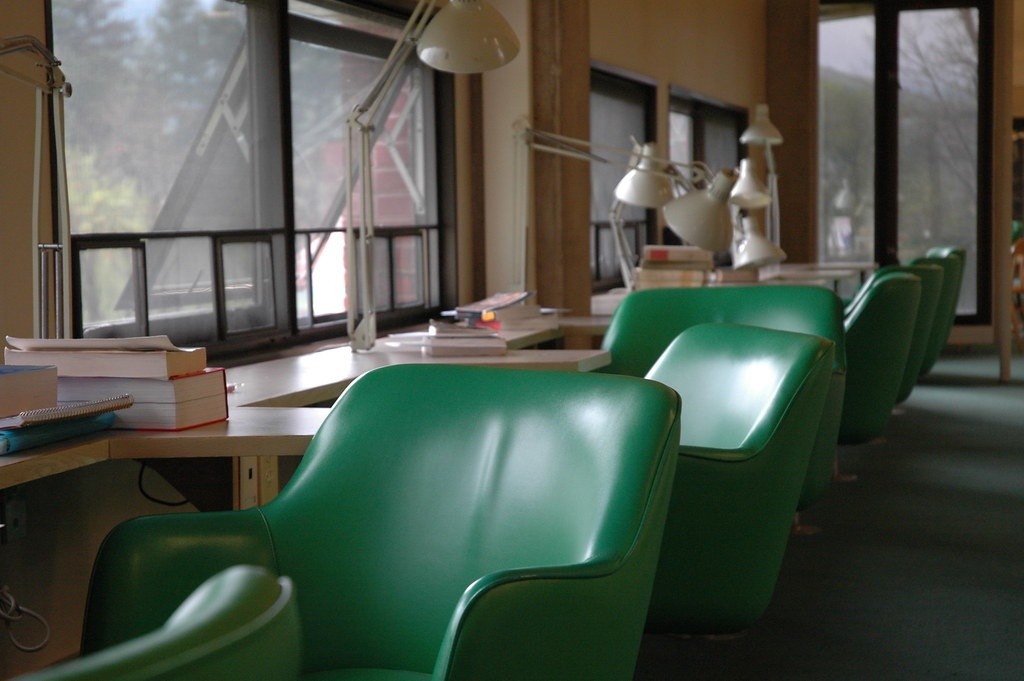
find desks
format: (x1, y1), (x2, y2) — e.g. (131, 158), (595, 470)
(0, 311), (616, 681)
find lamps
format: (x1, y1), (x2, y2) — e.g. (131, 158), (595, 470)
(730, 159), (773, 210)
(344, 0), (521, 353)
(729, 208), (787, 273)
(739, 102), (784, 275)
(608, 133), (675, 293)
(513, 117), (740, 250)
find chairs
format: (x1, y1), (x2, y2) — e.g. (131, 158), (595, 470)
(601, 284), (847, 511)
(7, 561), (302, 681)
(82, 361), (683, 681)
(639, 321), (842, 632)
(927, 245), (968, 351)
(910, 254), (960, 375)
(837, 272), (920, 447)
(843, 263), (944, 405)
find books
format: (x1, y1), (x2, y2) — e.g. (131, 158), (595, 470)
(455, 291), (559, 330)
(421, 318), (507, 357)
(632, 245), (761, 291)
(0, 334), (230, 455)
